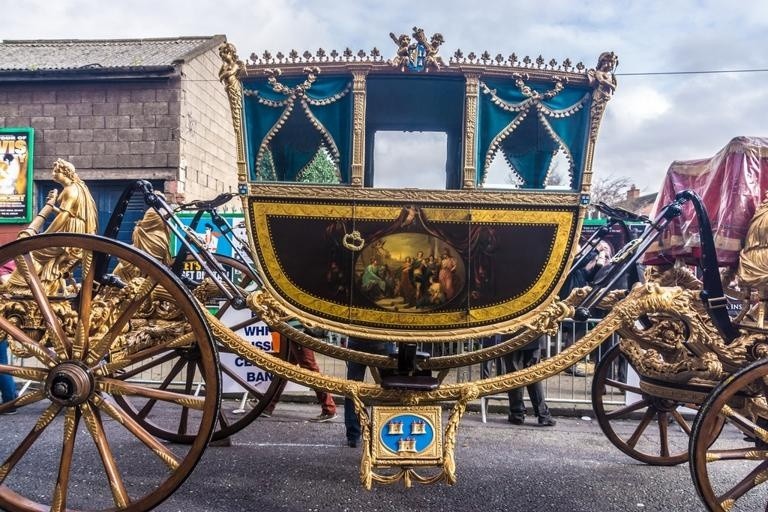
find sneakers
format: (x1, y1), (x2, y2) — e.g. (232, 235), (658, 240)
(507, 412), (525, 426)
(538, 416), (558, 426)
(246, 400), (273, 418)
(310, 413), (339, 423)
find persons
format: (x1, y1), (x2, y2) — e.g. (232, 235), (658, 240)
(196, 223), (218, 253)
(597, 51), (619, 94)
(219, 43), (240, 84)
(111, 190), (169, 278)
(1, 341), (18, 415)
(565, 241), (627, 395)
(10, 158), (97, 290)
(255, 292), (558, 448)
(397, 33), (445, 71)
(360, 248), (460, 304)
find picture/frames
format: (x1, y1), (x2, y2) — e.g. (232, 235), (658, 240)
(0, 126), (35, 226)
